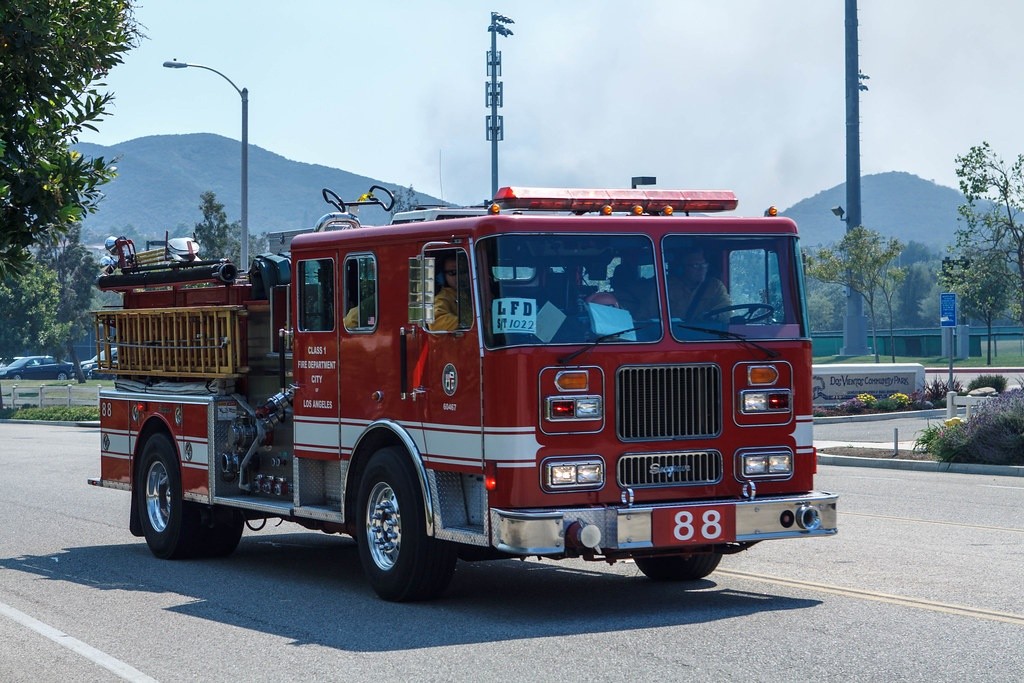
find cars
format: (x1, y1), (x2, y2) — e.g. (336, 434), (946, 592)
(79, 346), (119, 381)
(0, 355), (78, 384)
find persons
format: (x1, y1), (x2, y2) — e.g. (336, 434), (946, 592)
(343, 270), (375, 329)
(425, 250), (473, 331)
(667, 247), (735, 323)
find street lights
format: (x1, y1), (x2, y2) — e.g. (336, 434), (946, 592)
(162, 58), (249, 275)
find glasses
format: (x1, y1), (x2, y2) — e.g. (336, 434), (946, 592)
(445, 269), (466, 276)
(686, 262), (709, 269)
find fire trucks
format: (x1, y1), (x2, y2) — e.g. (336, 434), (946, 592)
(87, 190), (840, 603)
